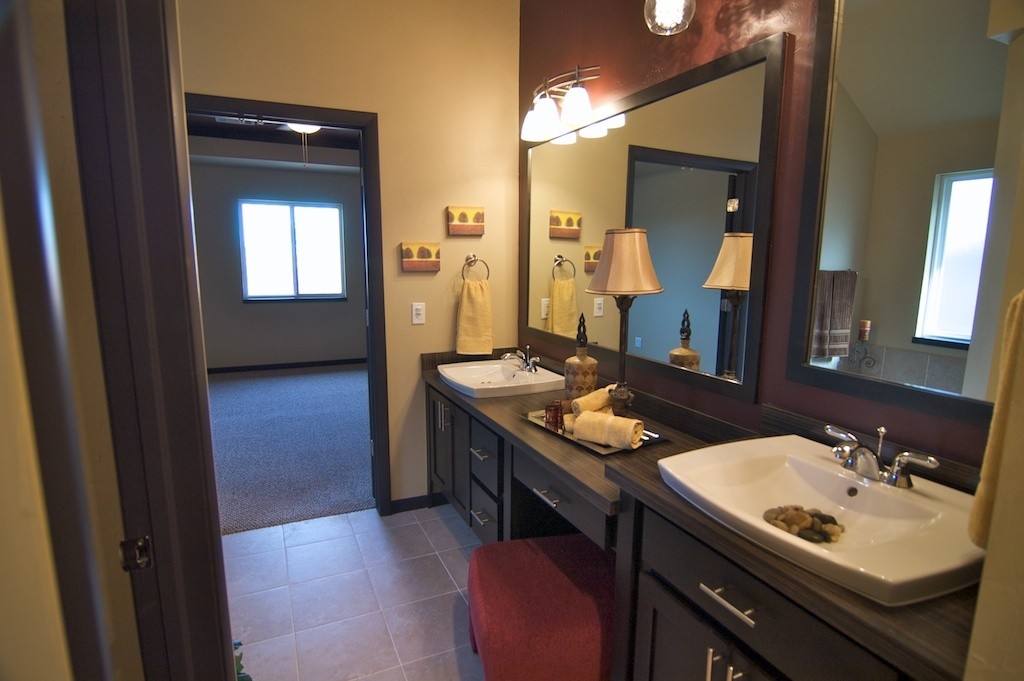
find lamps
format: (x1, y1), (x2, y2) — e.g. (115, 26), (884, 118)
(521, 64), (600, 143)
(644, 1), (697, 36)
(701, 233), (754, 384)
(585, 229), (664, 418)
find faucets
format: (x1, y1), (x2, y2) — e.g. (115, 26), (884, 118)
(500, 343), (540, 371)
(831, 423), (889, 479)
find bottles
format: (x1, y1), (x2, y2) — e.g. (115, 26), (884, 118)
(669, 308), (700, 372)
(551, 399), (564, 429)
(564, 312), (599, 401)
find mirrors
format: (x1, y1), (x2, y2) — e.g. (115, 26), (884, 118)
(783, 0), (1024, 428)
(518, 33), (789, 405)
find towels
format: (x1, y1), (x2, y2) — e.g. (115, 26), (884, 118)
(455, 280), (494, 356)
(811, 269), (860, 361)
(571, 383), (619, 416)
(573, 410), (645, 451)
(562, 405), (617, 432)
(545, 276), (577, 339)
(967, 290), (1023, 551)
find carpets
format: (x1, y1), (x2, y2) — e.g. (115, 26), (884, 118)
(205, 363), (376, 537)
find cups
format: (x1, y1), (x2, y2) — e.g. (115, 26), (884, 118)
(545, 405), (558, 427)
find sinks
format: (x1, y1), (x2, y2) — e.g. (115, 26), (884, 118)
(444, 365), (533, 389)
(657, 433), (996, 610)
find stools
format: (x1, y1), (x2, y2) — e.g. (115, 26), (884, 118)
(469, 531), (612, 681)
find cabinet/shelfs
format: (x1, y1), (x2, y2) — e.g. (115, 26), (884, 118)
(420, 381), (511, 545)
(611, 489), (912, 681)
